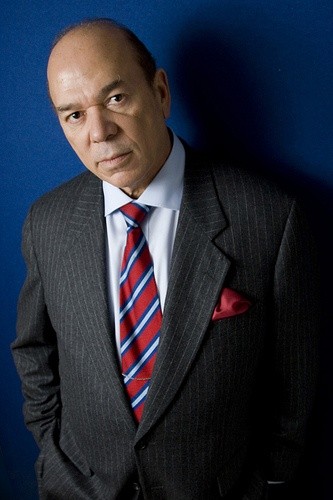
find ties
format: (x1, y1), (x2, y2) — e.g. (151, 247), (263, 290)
(118, 202), (162, 424)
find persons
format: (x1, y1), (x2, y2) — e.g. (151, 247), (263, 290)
(10, 18), (318, 500)
